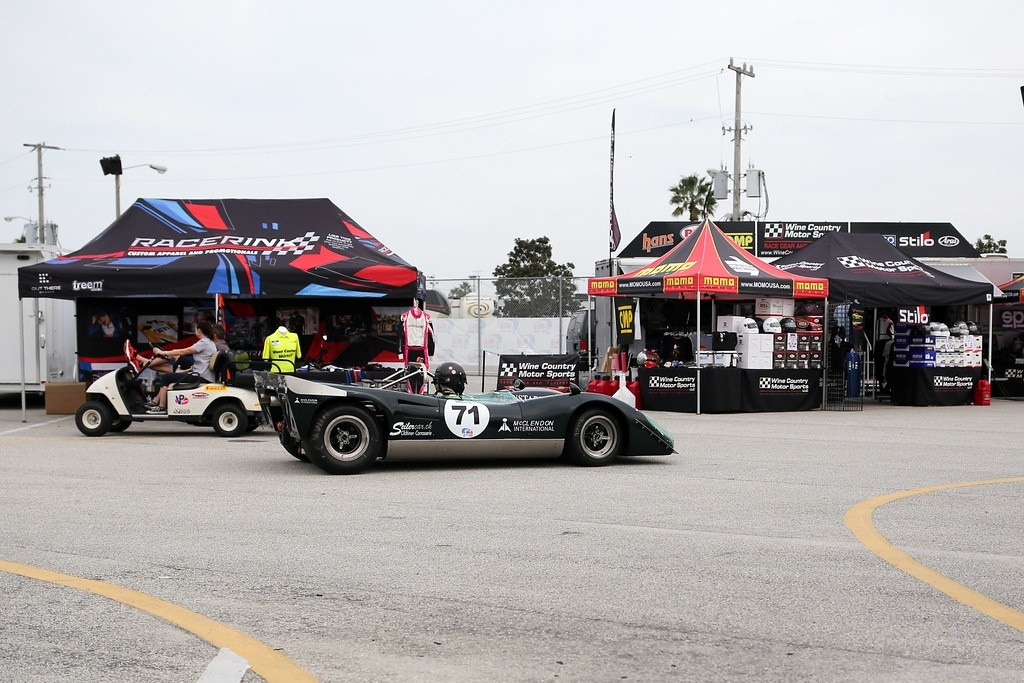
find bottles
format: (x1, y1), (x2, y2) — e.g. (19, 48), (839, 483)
(587, 375), (642, 409)
(974, 375), (991, 405)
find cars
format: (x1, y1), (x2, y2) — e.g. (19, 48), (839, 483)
(74, 332), (262, 438)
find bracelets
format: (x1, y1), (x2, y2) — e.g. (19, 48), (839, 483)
(165, 351), (166, 355)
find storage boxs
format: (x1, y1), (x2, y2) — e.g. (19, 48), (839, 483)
(718, 297), (825, 369)
(893, 325), (982, 368)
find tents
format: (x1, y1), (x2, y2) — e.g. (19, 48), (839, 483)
(768, 231), (994, 384)
(588, 218), (829, 414)
(996, 274), (1024, 303)
(18, 198), (427, 423)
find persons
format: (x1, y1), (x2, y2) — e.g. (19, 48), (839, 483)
(123, 324), (230, 373)
(143, 320), (217, 414)
(262, 326), (302, 372)
(433, 362), (468, 400)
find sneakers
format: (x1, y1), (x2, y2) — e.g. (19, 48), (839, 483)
(139, 401), (157, 410)
(130, 359), (142, 373)
(126, 339), (135, 360)
(146, 406), (167, 414)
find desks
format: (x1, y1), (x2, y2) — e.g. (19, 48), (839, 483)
(638, 366), (824, 415)
(890, 367), (983, 406)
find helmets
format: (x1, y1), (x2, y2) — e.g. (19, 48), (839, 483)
(949, 320), (969, 334)
(966, 320), (980, 334)
(739, 318), (759, 334)
(780, 318), (796, 333)
(939, 323), (950, 336)
(763, 317), (781, 333)
(433, 362), (467, 396)
(924, 322), (941, 336)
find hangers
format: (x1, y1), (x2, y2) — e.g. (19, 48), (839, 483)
(855, 299), (859, 304)
(850, 348), (855, 353)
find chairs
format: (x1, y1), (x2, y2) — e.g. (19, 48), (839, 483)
(406, 361), (428, 394)
(208, 349), (233, 383)
